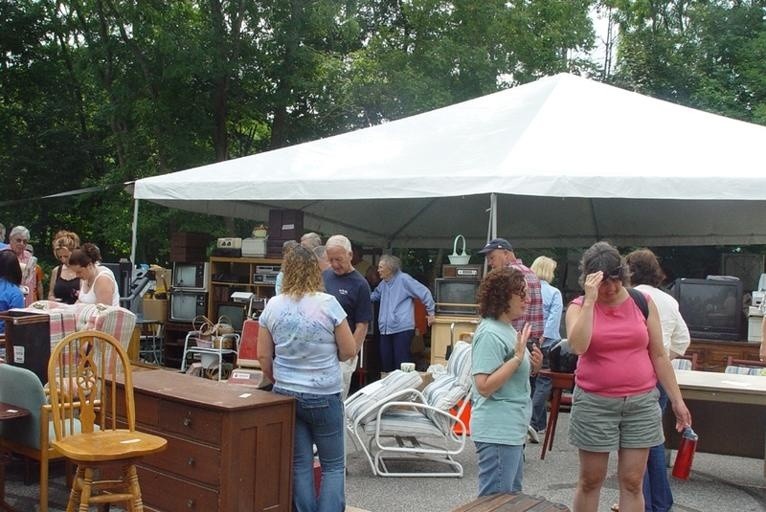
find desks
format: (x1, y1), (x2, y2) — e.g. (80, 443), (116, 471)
(537, 365), (576, 460)
(660, 368), (766, 478)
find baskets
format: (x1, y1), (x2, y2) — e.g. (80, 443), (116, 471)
(448, 234), (471, 265)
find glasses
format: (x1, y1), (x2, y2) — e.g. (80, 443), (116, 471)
(602, 264), (623, 281)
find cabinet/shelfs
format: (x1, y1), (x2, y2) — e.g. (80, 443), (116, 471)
(207, 256), (285, 330)
(430, 316), (479, 365)
(96, 368), (296, 512)
(684, 341), (761, 374)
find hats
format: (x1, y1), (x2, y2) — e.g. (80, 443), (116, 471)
(476, 237), (513, 254)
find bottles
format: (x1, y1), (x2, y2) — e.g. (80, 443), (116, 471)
(671, 424), (698, 480)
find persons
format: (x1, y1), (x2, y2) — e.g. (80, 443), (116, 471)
(370, 254), (436, 373)
(0, 222), (7, 250)
(321, 234), (371, 401)
(611, 246), (691, 512)
(256, 244), (357, 512)
(477, 237), (545, 399)
(4, 226), (37, 308)
(24, 244), (44, 301)
(275, 239), (299, 296)
(528, 256), (564, 443)
(69, 243), (121, 307)
(0, 249), (26, 335)
(468, 265), (545, 498)
(565, 242), (692, 512)
(48, 230), (84, 305)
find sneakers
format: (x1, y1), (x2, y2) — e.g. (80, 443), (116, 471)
(527, 425), (539, 444)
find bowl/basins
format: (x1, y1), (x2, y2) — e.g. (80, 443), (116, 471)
(401, 363), (415, 372)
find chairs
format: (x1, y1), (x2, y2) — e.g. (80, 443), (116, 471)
(344, 339), (475, 478)
(0, 363), (101, 512)
(47, 330), (168, 512)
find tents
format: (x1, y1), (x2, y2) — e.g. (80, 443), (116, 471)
(131, 72), (766, 284)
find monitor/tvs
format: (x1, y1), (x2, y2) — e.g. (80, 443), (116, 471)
(434, 278), (481, 317)
(169, 292), (208, 325)
(172, 262), (209, 292)
(674, 278), (743, 342)
(216, 303), (247, 333)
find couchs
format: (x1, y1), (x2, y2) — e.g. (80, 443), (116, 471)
(7, 299), (137, 400)
